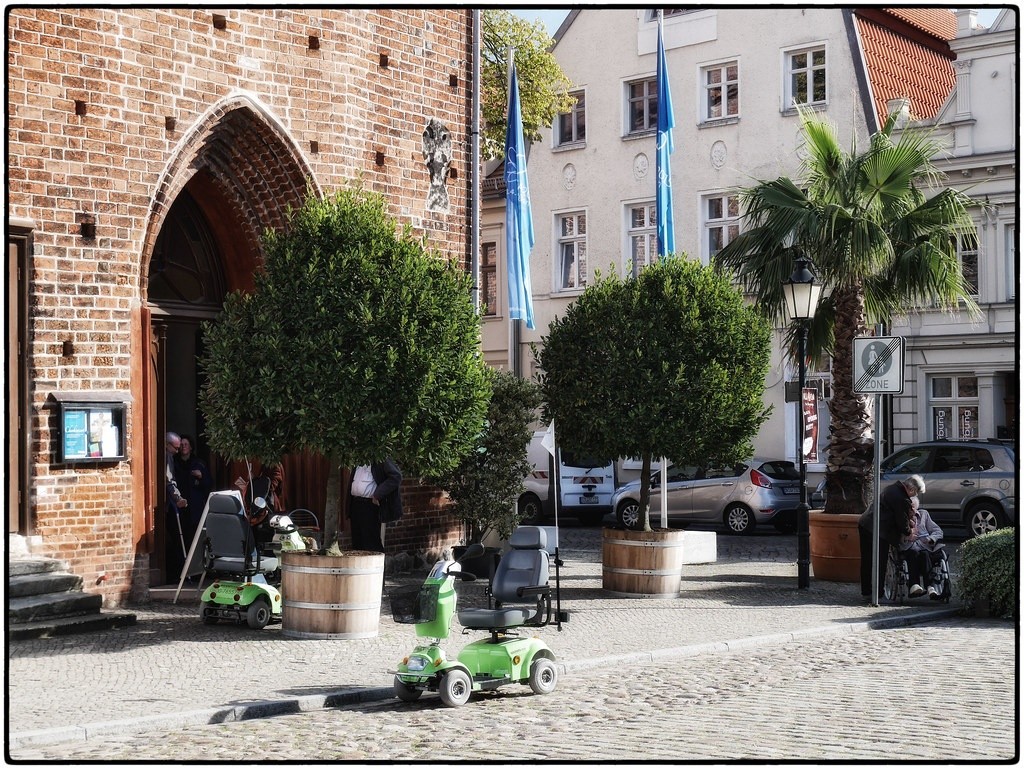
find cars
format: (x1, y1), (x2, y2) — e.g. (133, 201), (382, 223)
(605, 456), (798, 532)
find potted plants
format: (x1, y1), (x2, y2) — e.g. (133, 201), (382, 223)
(192, 98), (988, 642)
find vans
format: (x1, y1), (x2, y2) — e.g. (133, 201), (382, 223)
(513, 430), (617, 524)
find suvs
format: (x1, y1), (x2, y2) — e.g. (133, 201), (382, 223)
(813, 437), (1014, 538)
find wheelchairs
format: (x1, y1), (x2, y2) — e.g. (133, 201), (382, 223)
(886, 544), (951, 604)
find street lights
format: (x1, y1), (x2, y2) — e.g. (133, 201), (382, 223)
(781, 248), (824, 586)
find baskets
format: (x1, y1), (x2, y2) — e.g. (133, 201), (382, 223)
(298, 530), (322, 551)
(388, 584), (441, 624)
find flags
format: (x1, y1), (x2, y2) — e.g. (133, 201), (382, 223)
(657, 25), (674, 261)
(507, 66), (535, 328)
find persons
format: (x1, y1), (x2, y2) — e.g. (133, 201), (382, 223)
(901, 497), (943, 598)
(347, 458), (403, 551)
(235, 454), (286, 581)
(858, 475), (926, 597)
(165, 432), (210, 581)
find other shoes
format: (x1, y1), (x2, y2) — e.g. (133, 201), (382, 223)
(929, 588), (937, 598)
(910, 585), (923, 594)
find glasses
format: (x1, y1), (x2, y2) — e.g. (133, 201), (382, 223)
(169, 442), (180, 451)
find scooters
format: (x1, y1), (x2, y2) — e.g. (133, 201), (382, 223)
(387, 523), (570, 707)
(199, 493), (322, 629)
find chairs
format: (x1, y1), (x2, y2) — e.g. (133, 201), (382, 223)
(457, 527), (553, 635)
(203, 493), (278, 582)
